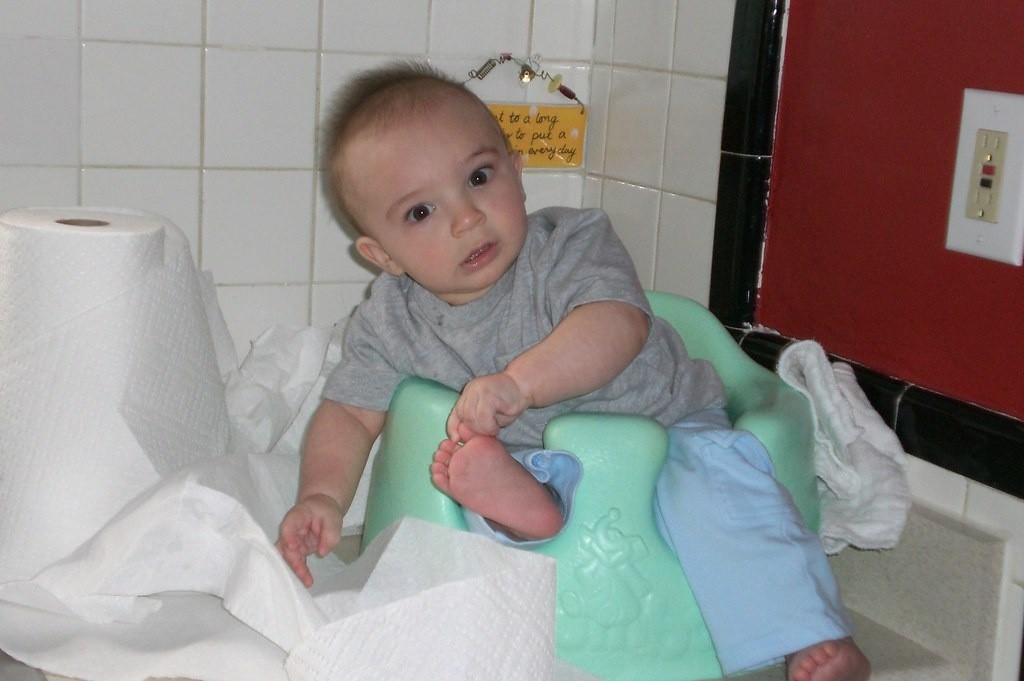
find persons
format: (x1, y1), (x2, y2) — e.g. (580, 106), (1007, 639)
(274, 59), (872, 681)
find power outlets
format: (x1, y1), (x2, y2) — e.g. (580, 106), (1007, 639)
(946, 86), (1024, 266)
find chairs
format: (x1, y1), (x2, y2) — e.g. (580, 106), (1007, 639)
(360, 287), (822, 681)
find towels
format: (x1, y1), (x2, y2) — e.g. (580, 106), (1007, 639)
(777, 340), (910, 556)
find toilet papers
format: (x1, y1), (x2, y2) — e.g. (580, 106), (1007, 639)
(0, 207), (164, 681)
